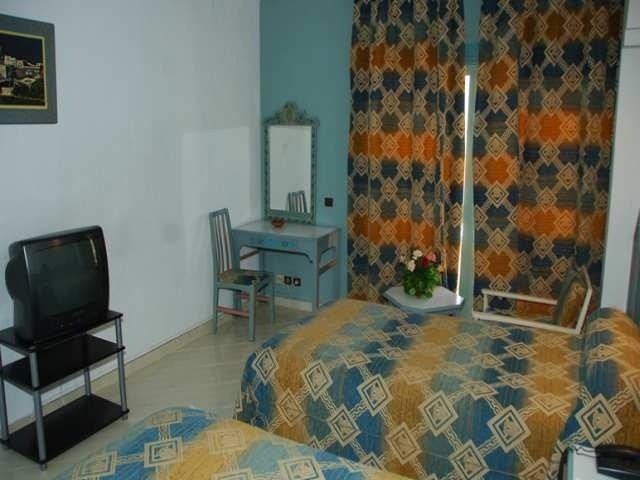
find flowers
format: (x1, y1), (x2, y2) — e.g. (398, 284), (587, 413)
(394, 247), (443, 299)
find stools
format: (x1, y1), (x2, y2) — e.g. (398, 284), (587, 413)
(382, 285), (466, 317)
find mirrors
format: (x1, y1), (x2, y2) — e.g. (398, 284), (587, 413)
(263, 101), (321, 226)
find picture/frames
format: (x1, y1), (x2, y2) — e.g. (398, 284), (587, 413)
(1, 14), (58, 124)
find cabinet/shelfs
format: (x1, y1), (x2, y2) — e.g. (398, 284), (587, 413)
(1, 309), (130, 472)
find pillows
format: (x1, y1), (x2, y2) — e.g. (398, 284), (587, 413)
(582, 306), (640, 450)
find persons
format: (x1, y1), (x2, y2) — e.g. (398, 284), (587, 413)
(15, 62), (30, 96)
(31, 66), (45, 99)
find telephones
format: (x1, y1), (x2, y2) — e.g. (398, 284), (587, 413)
(595, 443), (640, 480)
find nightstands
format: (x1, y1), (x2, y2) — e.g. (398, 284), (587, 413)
(562, 441), (624, 480)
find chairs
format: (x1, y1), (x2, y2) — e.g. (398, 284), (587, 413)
(471, 263), (594, 336)
(208, 208), (276, 342)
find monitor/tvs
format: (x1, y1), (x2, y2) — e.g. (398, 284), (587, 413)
(5, 226), (108, 344)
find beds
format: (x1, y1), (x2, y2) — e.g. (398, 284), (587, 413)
(53, 404), (415, 479)
(239, 297), (640, 480)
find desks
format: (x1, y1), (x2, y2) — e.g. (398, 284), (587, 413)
(231, 217), (342, 317)
